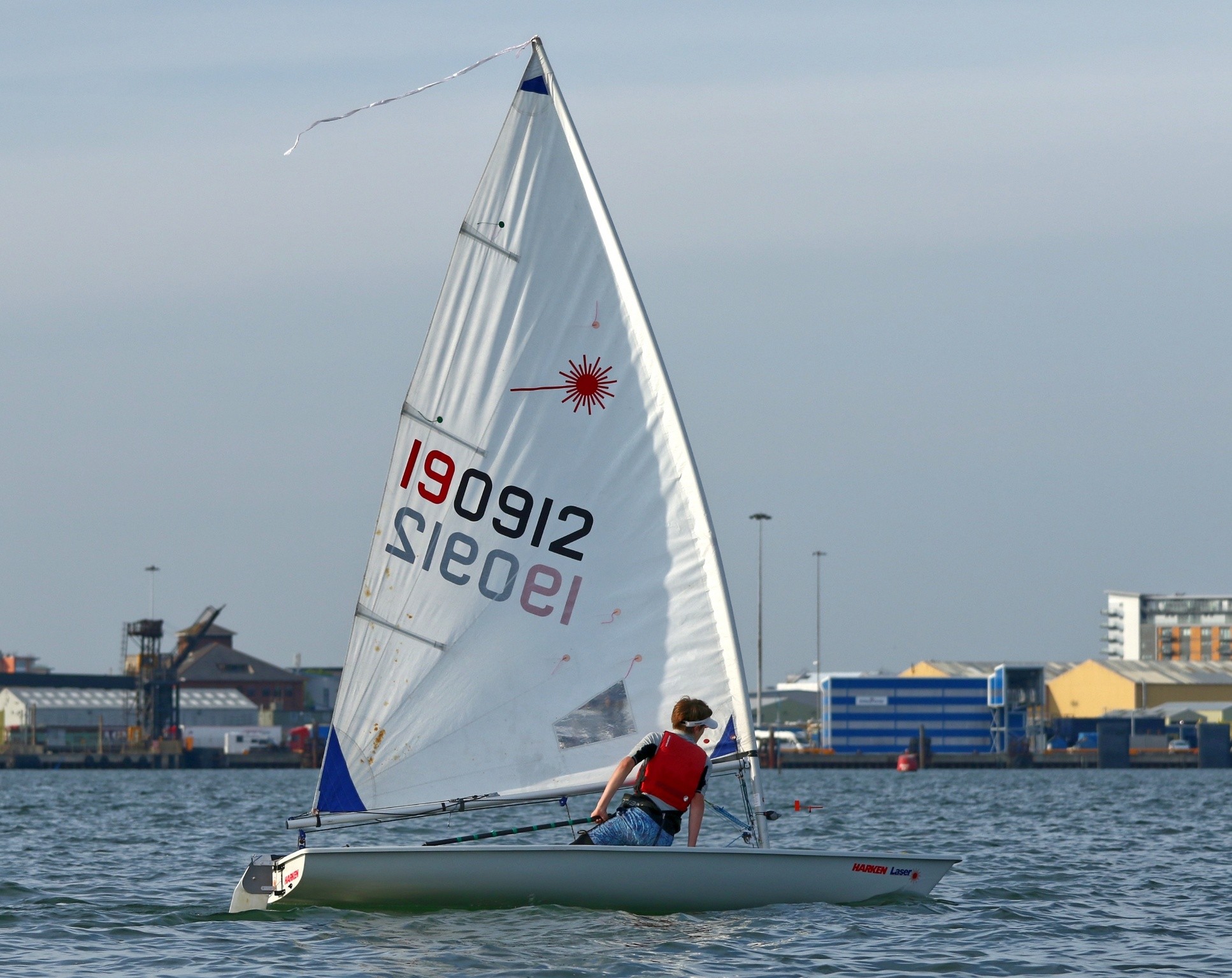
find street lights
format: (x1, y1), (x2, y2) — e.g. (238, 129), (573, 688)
(144, 565), (160, 620)
(812, 550), (828, 749)
(747, 513), (772, 729)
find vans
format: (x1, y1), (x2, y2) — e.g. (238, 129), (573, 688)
(224, 731), (280, 755)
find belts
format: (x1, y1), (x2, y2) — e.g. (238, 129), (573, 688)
(640, 804), (675, 836)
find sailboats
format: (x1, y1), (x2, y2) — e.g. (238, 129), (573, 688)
(228, 33), (965, 913)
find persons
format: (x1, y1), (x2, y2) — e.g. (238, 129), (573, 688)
(567, 695), (717, 847)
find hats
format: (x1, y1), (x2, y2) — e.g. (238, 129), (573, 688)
(680, 716), (718, 729)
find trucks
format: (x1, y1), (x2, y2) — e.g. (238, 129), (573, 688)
(288, 723), (331, 755)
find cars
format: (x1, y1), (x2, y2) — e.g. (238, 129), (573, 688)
(1167, 739), (1190, 750)
